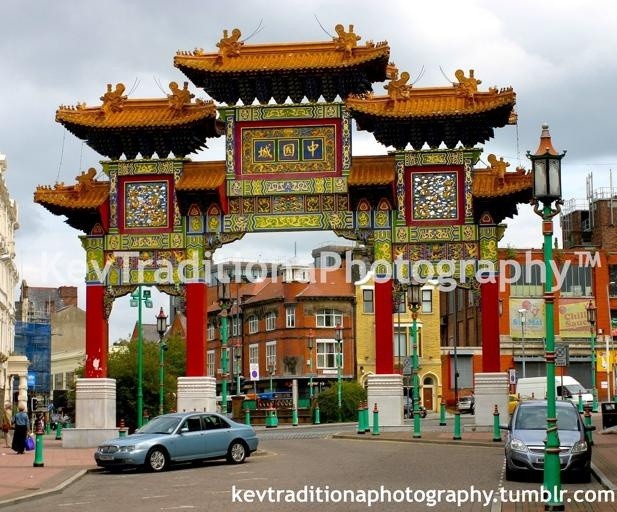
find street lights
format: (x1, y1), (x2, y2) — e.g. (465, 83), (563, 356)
(155, 306), (168, 414)
(236, 343), (241, 394)
(406, 269), (426, 437)
(334, 322), (345, 407)
(215, 273), (235, 419)
(130, 286), (153, 425)
(585, 299), (597, 412)
(526, 123), (568, 512)
(306, 329), (316, 398)
(268, 362), (275, 393)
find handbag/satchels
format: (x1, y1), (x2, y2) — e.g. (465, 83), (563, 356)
(25, 433), (35, 451)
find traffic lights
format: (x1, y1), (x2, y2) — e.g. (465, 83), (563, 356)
(33, 399), (38, 410)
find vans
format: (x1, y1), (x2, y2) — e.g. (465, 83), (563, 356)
(516, 376), (594, 411)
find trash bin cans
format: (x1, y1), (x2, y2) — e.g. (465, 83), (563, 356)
(601, 402), (617, 430)
(266, 409), (278, 428)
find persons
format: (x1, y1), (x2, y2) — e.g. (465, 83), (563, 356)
(469, 389), (475, 415)
(10, 404), (30, 455)
(2, 400), (12, 449)
(41, 416), (45, 433)
(63, 413), (69, 422)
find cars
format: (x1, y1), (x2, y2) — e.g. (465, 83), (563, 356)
(94, 411), (259, 472)
(505, 394), (591, 481)
(458, 395), (474, 414)
(404, 396), (427, 419)
(50, 414), (70, 430)
